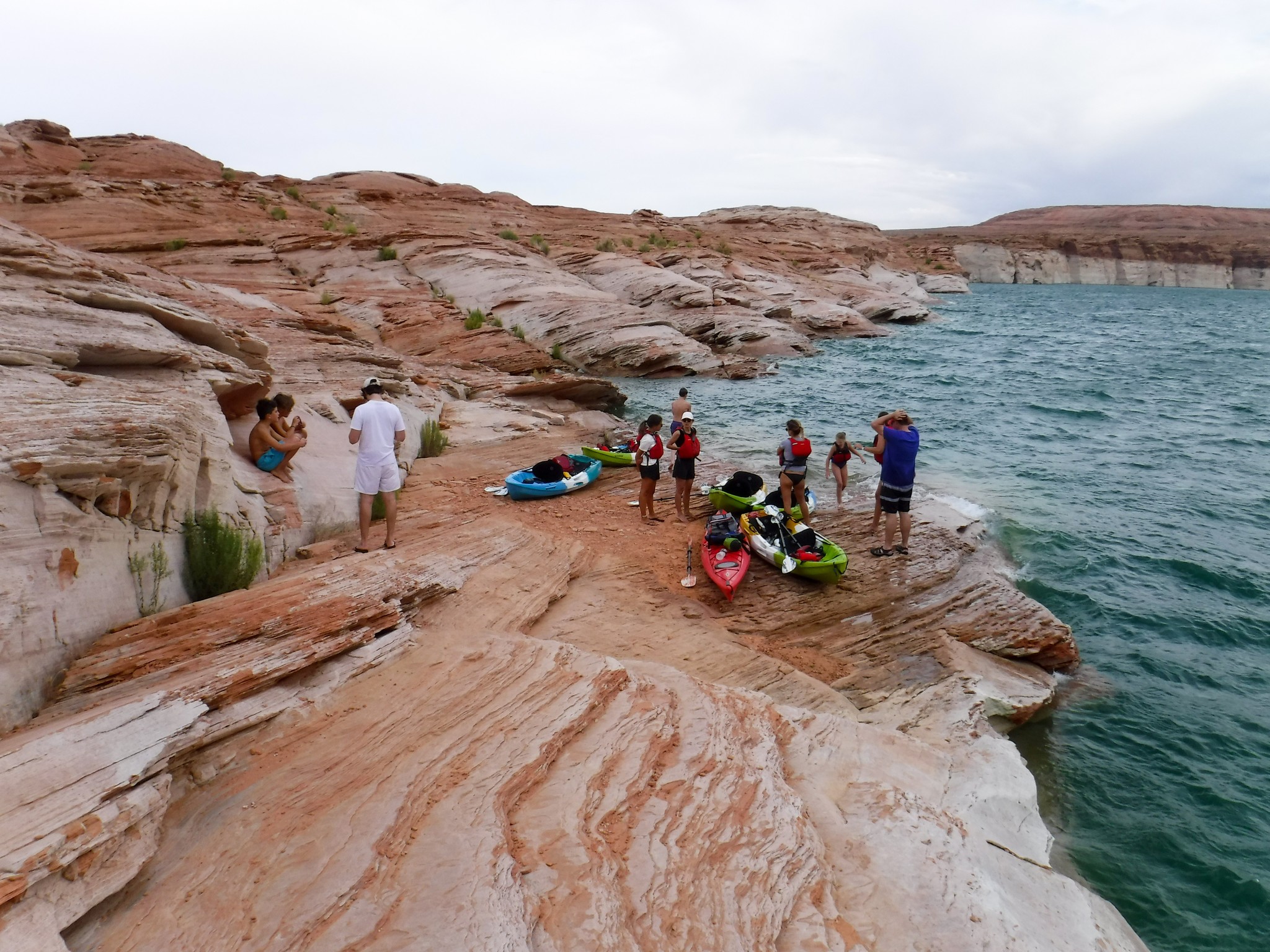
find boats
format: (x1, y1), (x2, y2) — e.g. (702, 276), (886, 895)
(752, 487), (817, 520)
(504, 455), (603, 499)
(580, 445), (637, 468)
(699, 509), (750, 603)
(739, 510), (847, 584)
(707, 470), (767, 514)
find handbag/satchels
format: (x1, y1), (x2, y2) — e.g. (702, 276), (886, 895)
(781, 528), (831, 554)
(747, 511), (796, 538)
(532, 455), (598, 483)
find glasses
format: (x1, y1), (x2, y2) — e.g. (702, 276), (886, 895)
(657, 424), (663, 427)
(785, 428), (789, 431)
(682, 418), (693, 422)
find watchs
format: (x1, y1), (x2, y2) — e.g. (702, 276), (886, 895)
(862, 446), (865, 450)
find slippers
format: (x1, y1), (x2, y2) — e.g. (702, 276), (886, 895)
(353, 546), (368, 553)
(384, 540), (396, 549)
(648, 517), (665, 522)
(644, 520), (658, 526)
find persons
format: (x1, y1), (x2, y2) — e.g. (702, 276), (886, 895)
(249, 393), (308, 484)
(825, 432), (866, 508)
(777, 419), (813, 530)
(666, 412), (699, 524)
(854, 411), (895, 533)
(635, 414), (665, 526)
(348, 377), (406, 553)
(870, 410), (920, 557)
(668, 387), (691, 469)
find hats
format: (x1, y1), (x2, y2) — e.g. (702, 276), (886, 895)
(681, 412), (694, 421)
(361, 377), (380, 389)
(888, 417), (907, 424)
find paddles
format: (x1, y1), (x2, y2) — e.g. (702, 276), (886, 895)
(493, 488), (508, 497)
(769, 513), (797, 576)
(680, 534), (697, 587)
(763, 503), (805, 549)
(484, 485), (507, 492)
(701, 482), (724, 495)
(626, 493), (710, 507)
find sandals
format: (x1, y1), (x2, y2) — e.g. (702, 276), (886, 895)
(870, 546), (893, 556)
(896, 544), (908, 555)
